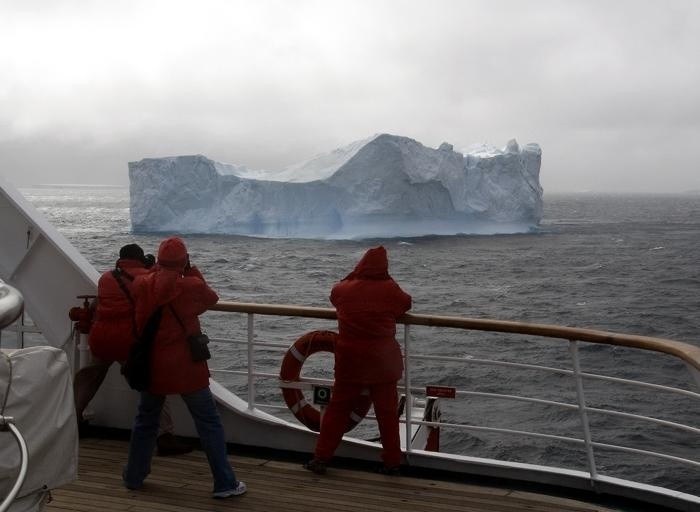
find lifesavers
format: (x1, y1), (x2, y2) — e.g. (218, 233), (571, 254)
(279, 330), (375, 433)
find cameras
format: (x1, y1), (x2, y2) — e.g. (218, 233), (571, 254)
(144, 254), (154, 268)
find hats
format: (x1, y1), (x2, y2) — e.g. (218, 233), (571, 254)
(159, 238), (187, 264)
(117, 242), (144, 258)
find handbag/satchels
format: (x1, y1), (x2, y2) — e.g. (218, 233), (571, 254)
(120, 305), (161, 391)
(169, 303), (211, 363)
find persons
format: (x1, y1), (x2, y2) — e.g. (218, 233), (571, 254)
(53, 242), (195, 459)
(302, 245), (414, 479)
(120, 236), (248, 499)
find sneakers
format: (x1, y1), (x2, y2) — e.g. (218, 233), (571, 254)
(303, 460), (327, 475)
(213, 480), (248, 499)
